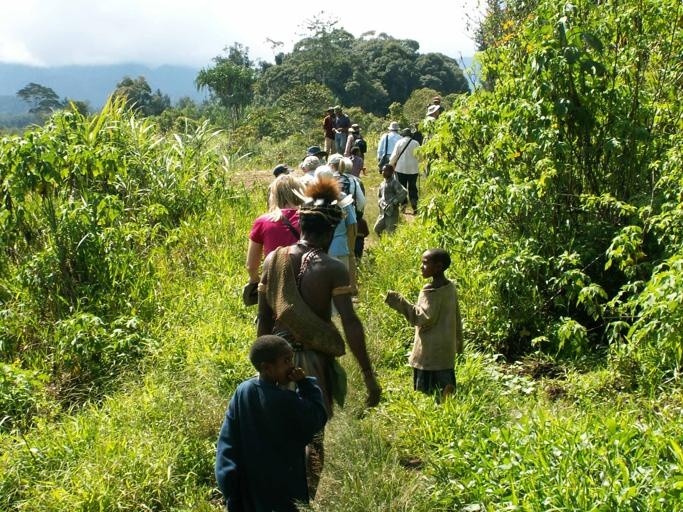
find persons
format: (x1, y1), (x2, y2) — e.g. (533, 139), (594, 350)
(258, 204), (382, 500)
(373, 97), (445, 239)
(214, 334), (328, 512)
(384, 248), (463, 404)
(242, 104), (370, 284)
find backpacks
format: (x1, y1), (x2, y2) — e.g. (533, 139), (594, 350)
(354, 137), (367, 153)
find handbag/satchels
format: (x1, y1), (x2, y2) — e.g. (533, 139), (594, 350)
(379, 200), (393, 217)
(326, 358), (347, 409)
(379, 155), (390, 174)
(358, 219), (369, 239)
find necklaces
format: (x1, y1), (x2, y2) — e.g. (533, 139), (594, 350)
(296, 239), (323, 279)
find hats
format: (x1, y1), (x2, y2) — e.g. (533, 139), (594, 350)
(296, 175), (343, 227)
(327, 97), (441, 136)
(302, 147), (343, 164)
(274, 165), (287, 176)
